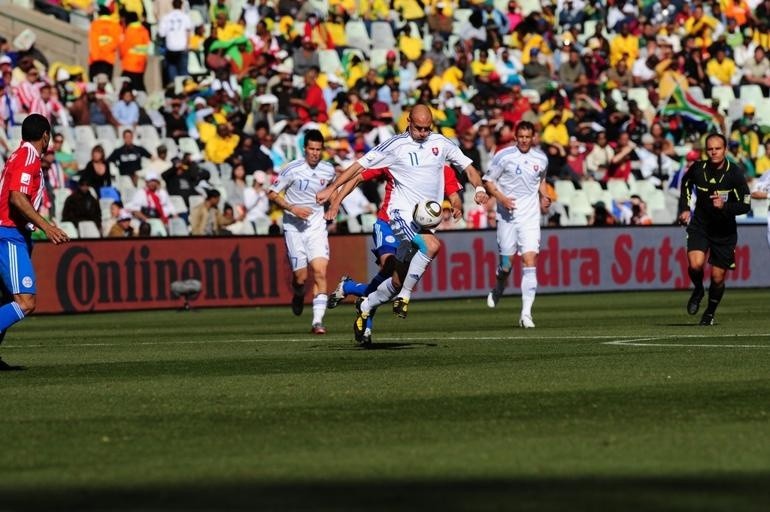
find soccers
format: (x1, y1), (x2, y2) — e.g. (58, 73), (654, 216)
(413, 199), (443, 229)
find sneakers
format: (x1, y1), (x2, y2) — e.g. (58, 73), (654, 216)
(354, 296), (370, 337)
(687, 288), (704, 315)
(312, 323), (326, 334)
(327, 276), (352, 309)
(293, 294), (304, 315)
(393, 297), (409, 319)
(519, 316), (535, 328)
(487, 287), (504, 308)
(700, 312), (714, 326)
(356, 328), (371, 346)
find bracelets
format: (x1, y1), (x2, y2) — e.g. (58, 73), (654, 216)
(475, 186), (486, 193)
(283, 202), (292, 210)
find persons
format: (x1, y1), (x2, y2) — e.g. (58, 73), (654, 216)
(676, 132), (752, 327)
(480, 120), (551, 329)
(267, 129), (339, 333)
(321, 160), (464, 341)
(315, 103), (488, 342)
(0, 0), (770, 241)
(0, 112), (71, 371)
